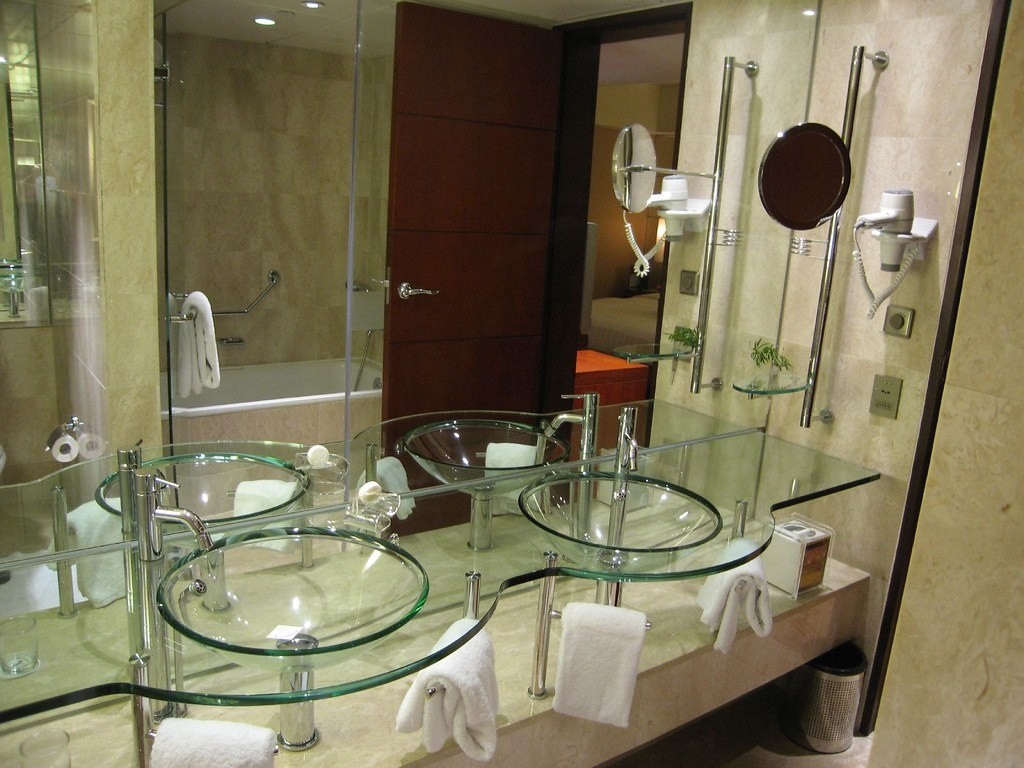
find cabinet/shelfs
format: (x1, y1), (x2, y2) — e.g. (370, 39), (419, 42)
(570, 350), (648, 485)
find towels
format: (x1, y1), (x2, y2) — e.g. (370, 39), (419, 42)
(393, 617), (500, 763)
(45, 495), (129, 610)
(178, 288), (223, 401)
(233, 477), (298, 519)
(147, 716), (279, 768)
(552, 601), (646, 729)
(696, 538), (775, 654)
(356, 454), (416, 522)
(484, 440), (542, 507)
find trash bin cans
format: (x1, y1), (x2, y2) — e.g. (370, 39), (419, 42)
(785, 637), (868, 754)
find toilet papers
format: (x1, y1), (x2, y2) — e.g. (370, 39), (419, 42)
(52, 434), (79, 463)
(77, 432), (107, 459)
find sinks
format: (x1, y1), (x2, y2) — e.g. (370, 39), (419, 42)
(92, 447), (310, 527)
(516, 468), (726, 554)
(155, 521), (435, 662)
(404, 417), (571, 501)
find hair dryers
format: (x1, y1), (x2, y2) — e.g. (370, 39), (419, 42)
(660, 176), (689, 242)
(873, 187), (916, 273)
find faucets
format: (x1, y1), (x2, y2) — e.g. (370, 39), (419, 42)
(615, 404), (639, 473)
(132, 467), (215, 562)
(544, 391), (602, 454)
(116, 437), (173, 542)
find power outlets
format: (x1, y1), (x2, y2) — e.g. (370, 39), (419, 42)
(870, 374), (903, 420)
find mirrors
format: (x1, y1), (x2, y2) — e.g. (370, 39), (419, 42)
(759, 122), (851, 230)
(611, 122), (714, 213)
(0, 0), (825, 576)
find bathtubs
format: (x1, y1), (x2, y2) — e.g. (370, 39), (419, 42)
(169, 354), (382, 516)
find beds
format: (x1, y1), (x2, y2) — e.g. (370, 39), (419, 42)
(587, 292), (660, 366)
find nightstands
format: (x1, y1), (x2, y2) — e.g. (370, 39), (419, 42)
(623, 280), (659, 298)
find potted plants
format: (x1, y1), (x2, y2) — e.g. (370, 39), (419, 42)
(752, 339), (794, 387)
(669, 327), (697, 350)
